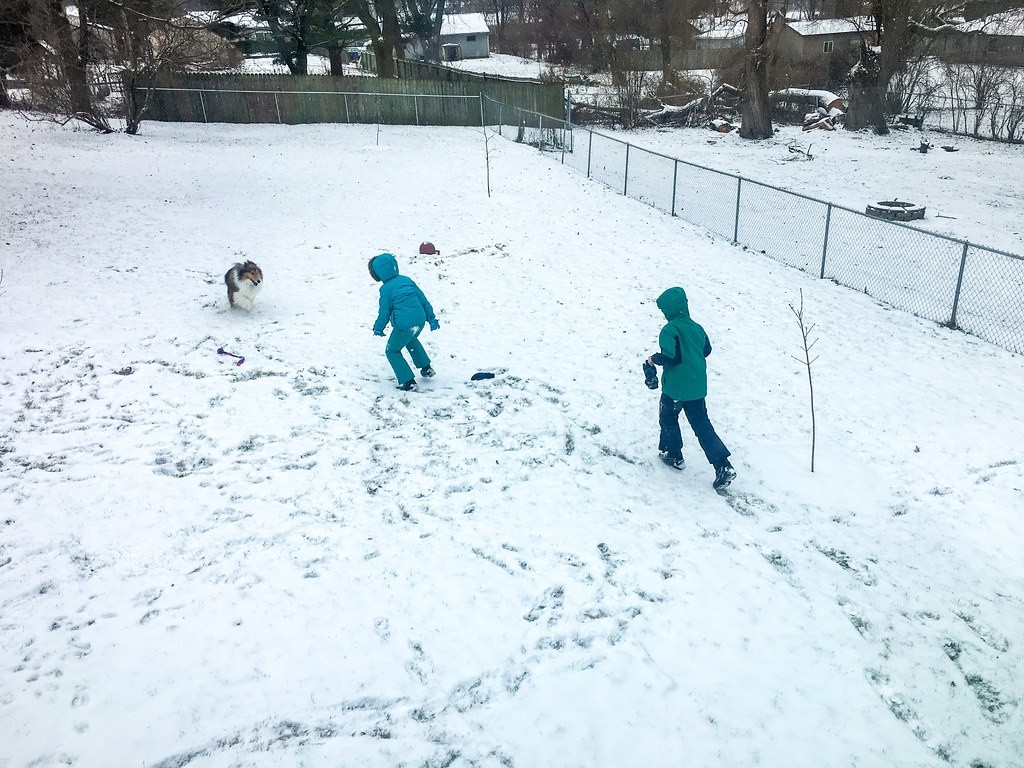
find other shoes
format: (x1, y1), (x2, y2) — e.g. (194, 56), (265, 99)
(658, 451), (685, 470)
(420, 368), (436, 378)
(396, 379), (419, 392)
(713, 457), (736, 489)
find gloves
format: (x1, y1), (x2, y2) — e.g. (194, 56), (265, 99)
(642, 360), (658, 389)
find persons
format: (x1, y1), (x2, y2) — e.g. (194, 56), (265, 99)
(649, 287), (736, 490)
(368, 253), (440, 392)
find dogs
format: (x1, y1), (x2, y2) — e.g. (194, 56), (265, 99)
(224, 259), (263, 311)
